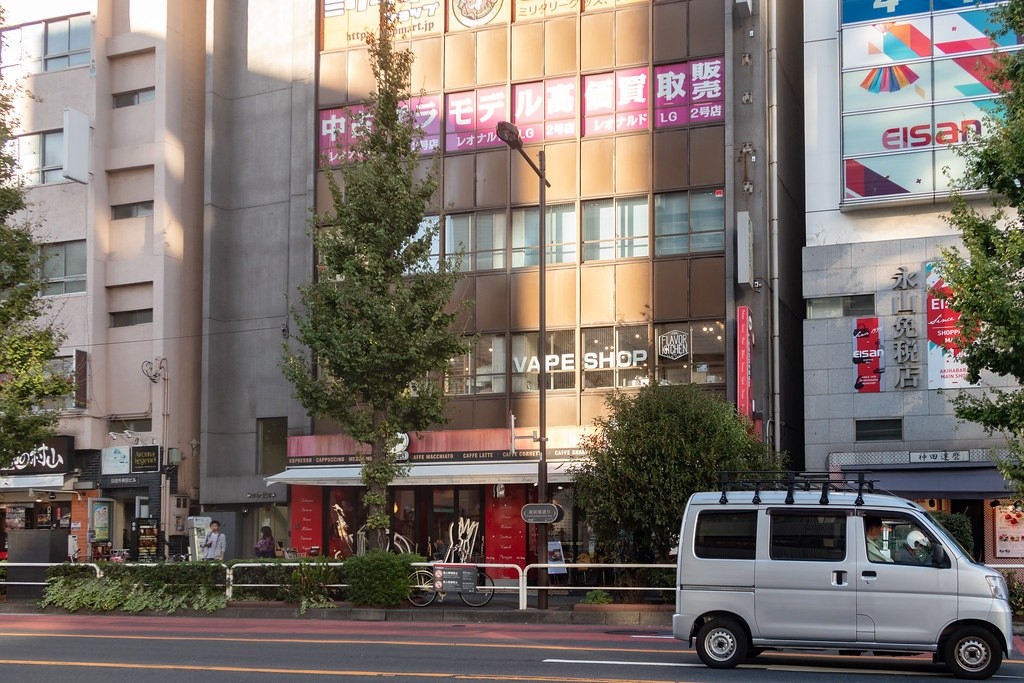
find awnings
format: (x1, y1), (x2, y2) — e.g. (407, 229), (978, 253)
(0, 471), (79, 490)
(263, 462), (597, 484)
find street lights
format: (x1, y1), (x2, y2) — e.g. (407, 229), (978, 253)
(495, 120), (550, 611)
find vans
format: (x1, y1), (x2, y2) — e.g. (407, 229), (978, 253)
(672, 469), (1013, 681)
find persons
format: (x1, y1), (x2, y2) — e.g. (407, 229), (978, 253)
(255, 526), (277, 559)
(864, 517), (892, 563)
(200, 521), (227, 564)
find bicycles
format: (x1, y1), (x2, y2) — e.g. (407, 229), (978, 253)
(406, 547), (495, 608)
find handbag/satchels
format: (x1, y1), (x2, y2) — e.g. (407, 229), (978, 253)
(275, 538), (285, 558)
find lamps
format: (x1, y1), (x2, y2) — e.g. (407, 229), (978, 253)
(28, 488), (35, 497)
(124, 430), (132, 438)
(109, 432), (116, 440)
